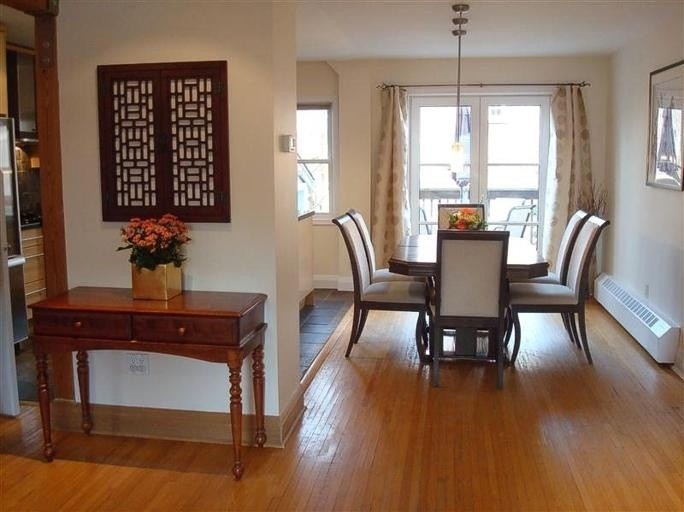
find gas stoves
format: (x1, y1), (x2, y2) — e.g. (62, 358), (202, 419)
(19, 210), (44, 231)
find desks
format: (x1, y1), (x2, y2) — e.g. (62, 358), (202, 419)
(28, 287), (268, 479)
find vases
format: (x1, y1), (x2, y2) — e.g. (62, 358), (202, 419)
(130, 263), (182, 300)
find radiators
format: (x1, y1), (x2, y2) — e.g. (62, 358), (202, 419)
(593, 273), (680, 364)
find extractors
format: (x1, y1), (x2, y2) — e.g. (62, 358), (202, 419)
(5, 51), (39, 148)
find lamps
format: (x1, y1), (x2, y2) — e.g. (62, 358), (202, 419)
(448, 31), (467, 175)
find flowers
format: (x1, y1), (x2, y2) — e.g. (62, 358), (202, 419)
(115, 215), (192, 271)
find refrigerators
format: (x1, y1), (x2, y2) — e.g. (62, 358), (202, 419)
(0, 117), (29, 353)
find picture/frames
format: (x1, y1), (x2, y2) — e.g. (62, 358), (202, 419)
(646, 62), (683, 192)
